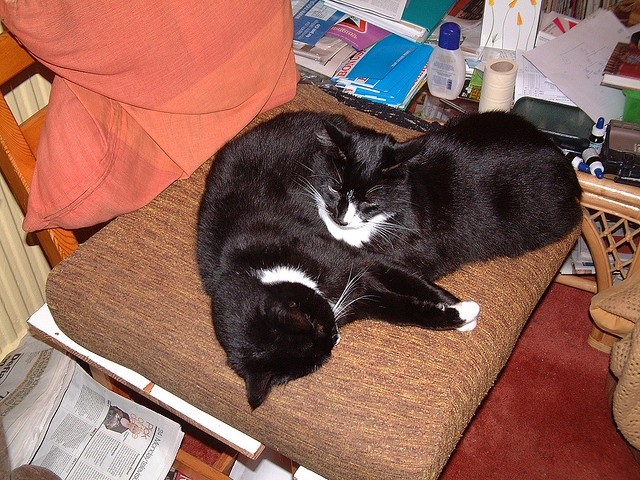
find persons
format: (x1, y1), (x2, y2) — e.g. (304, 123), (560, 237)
(103, 405), (138, 436)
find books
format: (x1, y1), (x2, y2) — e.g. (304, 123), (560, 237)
(599, 42), (640, 92)
(292, 0), (455, 112)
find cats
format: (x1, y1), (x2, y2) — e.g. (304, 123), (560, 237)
(195, 111), (479, 412)
(282, 109), (586, 282)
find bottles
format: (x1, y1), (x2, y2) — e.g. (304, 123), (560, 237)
(562, 150), (590, 172)
(590, 117), (607, 154)
(425, 23), (466, 99)
(582, 147), (604, 180)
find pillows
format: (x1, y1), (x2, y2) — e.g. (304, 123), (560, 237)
(1, 0), (301, 232)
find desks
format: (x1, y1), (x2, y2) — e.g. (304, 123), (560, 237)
(571, 167), (640, 357)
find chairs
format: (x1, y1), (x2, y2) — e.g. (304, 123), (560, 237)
(0, 27), (584, 480)
